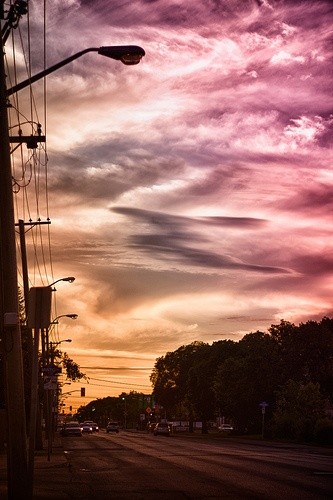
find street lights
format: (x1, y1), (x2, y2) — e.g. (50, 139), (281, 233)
(0, 44), (146, 500)
(28, 276), (75, 435)
(259, 402), (268, 441)
(40, 313), (72, 465)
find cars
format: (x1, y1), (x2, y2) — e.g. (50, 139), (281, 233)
(92, 424), (99, 433)
(63, 422), (83, 436)
(83, 423), (92, 434)
(218, 424), (233, 431)
(147, 421), (156, 432)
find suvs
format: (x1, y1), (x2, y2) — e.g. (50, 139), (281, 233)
(153, 422), (170, 437)
(106, 421), (120, 433)
